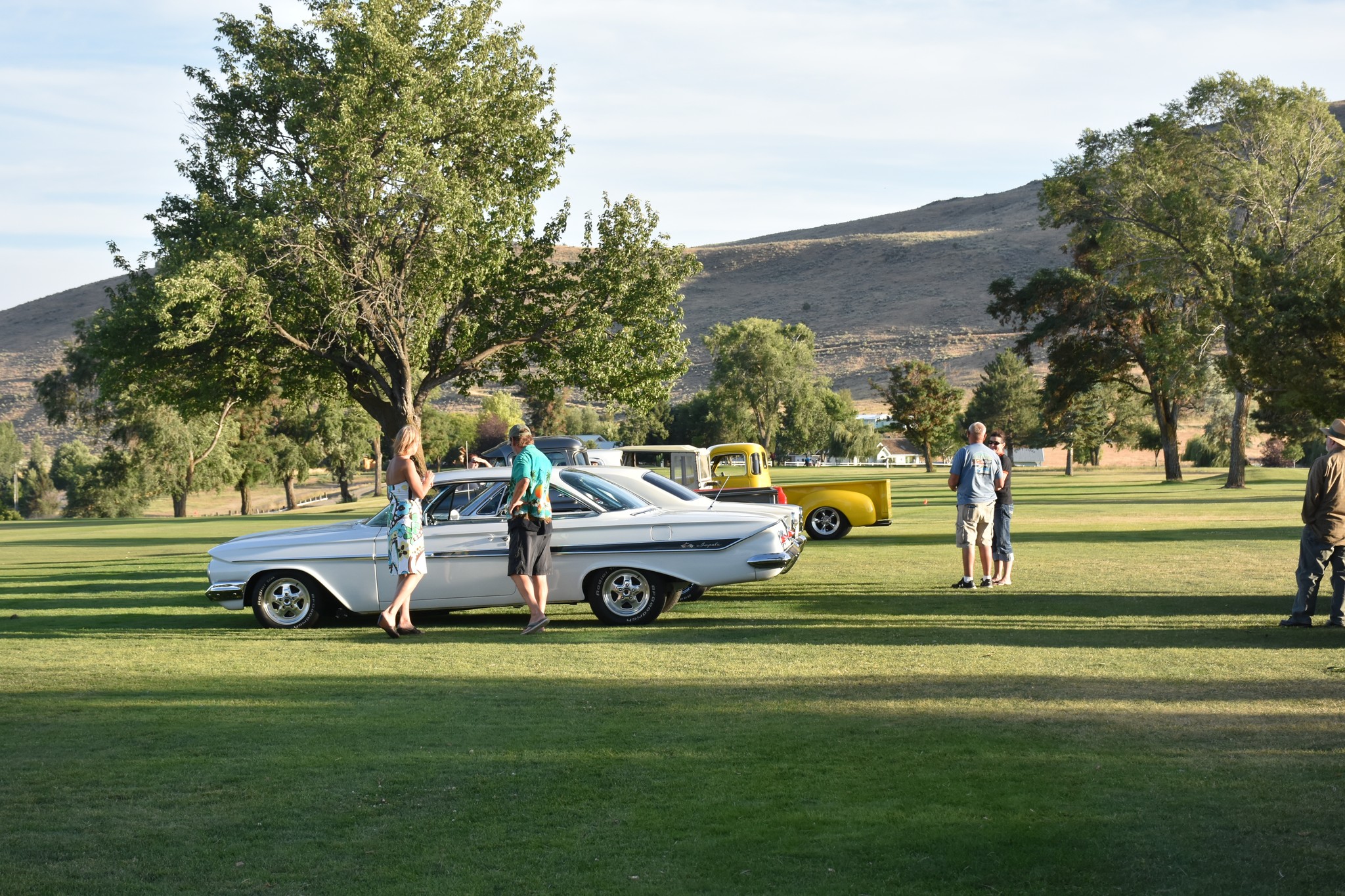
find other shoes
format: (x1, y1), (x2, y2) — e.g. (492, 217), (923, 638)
(1279, 620), (1312, 627)
(1324, 619), (1345, 628)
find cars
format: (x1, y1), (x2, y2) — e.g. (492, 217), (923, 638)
(203, 466), (808, 629)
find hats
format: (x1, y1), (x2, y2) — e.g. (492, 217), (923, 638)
(506, 423), (532, 445)
(1318, 418), (1345, 446)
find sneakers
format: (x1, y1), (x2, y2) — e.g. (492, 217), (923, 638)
(951, 577), (977, 590)
(980, 577), (993, 588)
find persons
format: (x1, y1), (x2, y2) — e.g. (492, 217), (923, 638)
(377, 426), (435, 638)
(453, 453), (493, 491)
(989, 430), (1014, 585)
(1278, 418), (1345, 627)
(947, 421), (1004, 591)
(768, 452), (776, 467)
(803, 458), (811, 467)
(506, 423), (553, 636)
(453, 447), (466, 466)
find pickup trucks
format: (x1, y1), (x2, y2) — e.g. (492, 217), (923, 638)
(481, 418), (893, 541)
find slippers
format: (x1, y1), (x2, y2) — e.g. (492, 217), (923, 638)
(377, 614), (400, 639)
(991, 580), (1010, 586)
(520, 618), (550, 636)
(396, 625), (425, 635)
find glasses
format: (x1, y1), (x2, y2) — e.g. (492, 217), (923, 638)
(509, 443), (511, 447)
(1325, 435), (1327, 438)
(470, 461), (479, 464)
(988, 440), (1004, 445)
